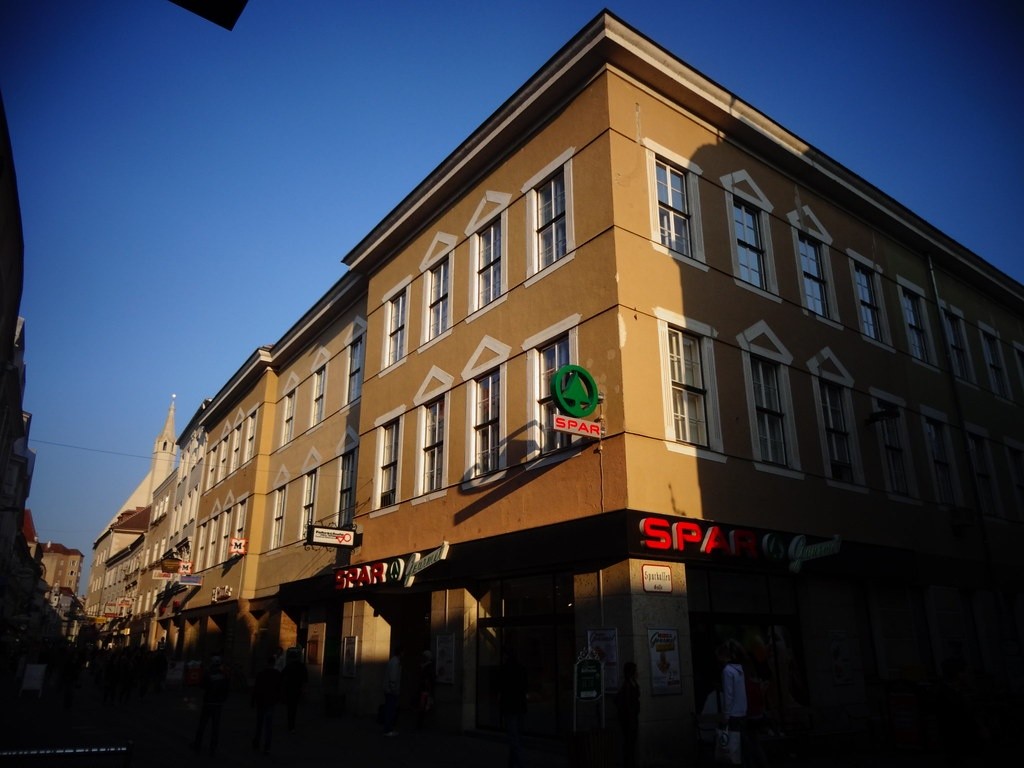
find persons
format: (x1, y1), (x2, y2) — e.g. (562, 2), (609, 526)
(7, 639), (168, 706)
(489, 643), (530, 768)
(716, 639), (766, 768)
(614, 662), (640, 768)
(280, 651), (309, 734)
(381, 646), (407, 737)
(252, 657), (279, 754)
(189, 662), (230, 757)
(414, 651), (434, 731)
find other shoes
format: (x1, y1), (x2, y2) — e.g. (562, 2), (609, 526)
(383, 729), (400, 737)
(290, 729), (296, 735)
(208, 748), (216, 756)
(252, 737), (259, 751)
(265, 748), (272, 753)
(190, 742), (200, 757)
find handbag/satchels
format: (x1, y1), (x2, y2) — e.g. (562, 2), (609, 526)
(714, 725), (742, 765)
(376, 704), (400, 726)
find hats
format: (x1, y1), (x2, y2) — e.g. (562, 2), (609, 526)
(211, 655), (222, 664)
